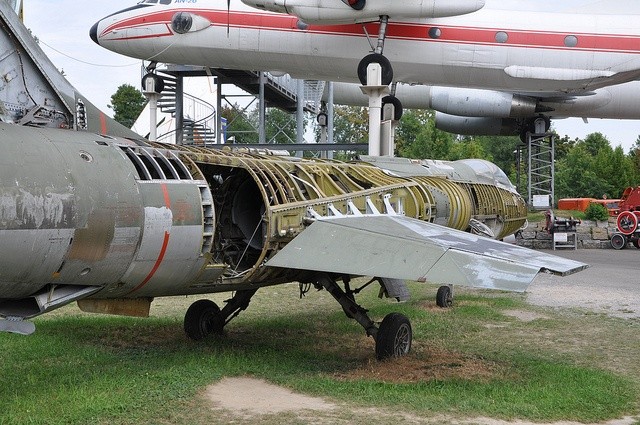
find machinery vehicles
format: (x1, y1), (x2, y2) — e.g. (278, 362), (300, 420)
(611, 186), (640, 249)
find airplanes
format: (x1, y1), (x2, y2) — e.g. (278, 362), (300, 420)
(0, 1), (590, 358)
(88, 1), (639, 138)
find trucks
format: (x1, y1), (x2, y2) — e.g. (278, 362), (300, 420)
(558, 198), (619, 216)
